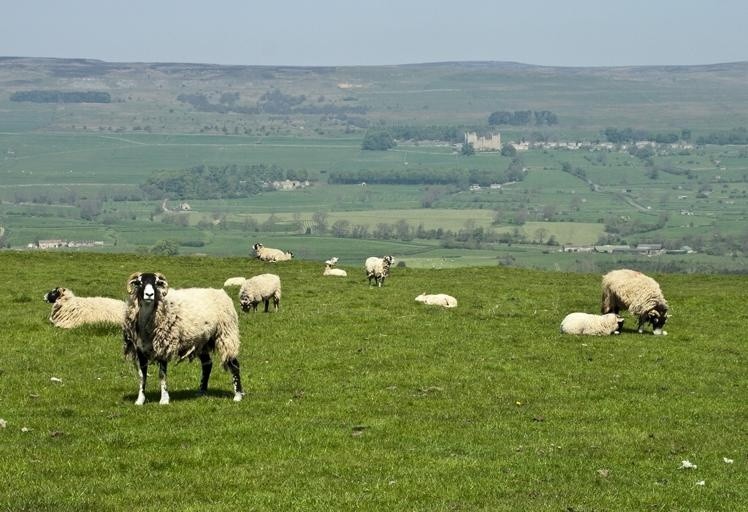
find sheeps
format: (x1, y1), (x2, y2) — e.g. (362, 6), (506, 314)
(238, 274), (281, 313)
(322, 257), (348, 277)
(600, 268), (673, 336)
(122, 271), (245, 406)
(252, 242), (294, 263)
(560, 312), (625, 337)
(414, 292), (458, 308)
(224, 277), (246, 287)
(364, 255), (395, 287)
(42, 286), (126, 329)
